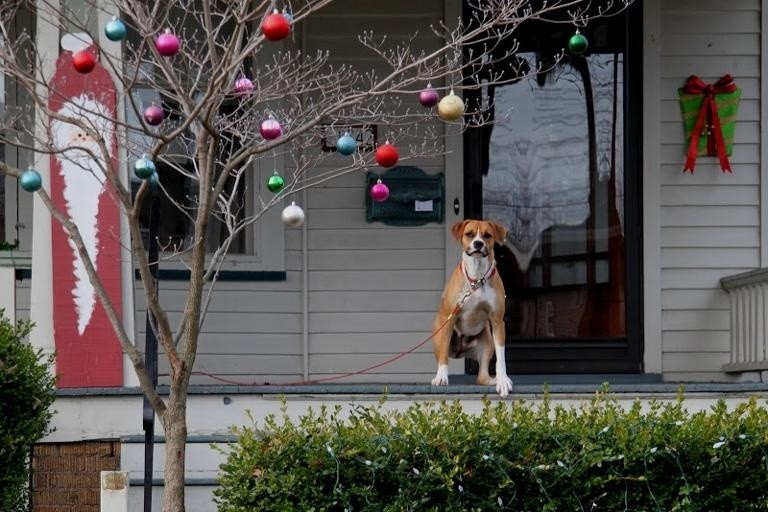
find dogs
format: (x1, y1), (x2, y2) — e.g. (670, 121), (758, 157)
(431, 219), (514, 400)
